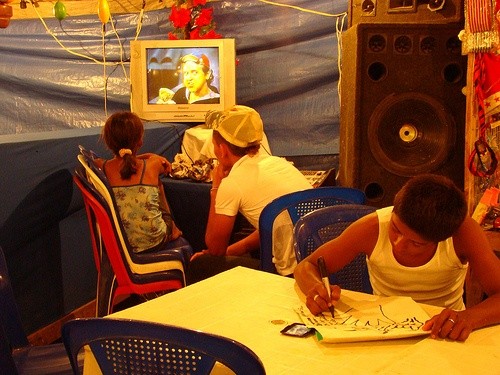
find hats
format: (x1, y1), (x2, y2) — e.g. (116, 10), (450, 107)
(206, 105), (263, 147)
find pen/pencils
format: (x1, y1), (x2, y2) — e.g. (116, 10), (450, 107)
(317, 255), (335, 318)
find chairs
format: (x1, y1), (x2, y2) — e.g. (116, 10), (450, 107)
(60, 143), (379, 375)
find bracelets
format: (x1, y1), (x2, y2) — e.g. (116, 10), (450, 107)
(210, 186), (218, 191)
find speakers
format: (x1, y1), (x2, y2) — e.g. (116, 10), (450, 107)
(338, 20), (465, 216)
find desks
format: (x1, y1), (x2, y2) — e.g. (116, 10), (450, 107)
(161, 175), (243, 250)
(102, 266), (500, 375)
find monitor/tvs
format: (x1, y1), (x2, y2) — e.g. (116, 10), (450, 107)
(129, 39), (236, 123)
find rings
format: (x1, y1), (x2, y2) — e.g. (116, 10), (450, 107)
(448, 318), (455, 324)
(314, 294), (320, 301)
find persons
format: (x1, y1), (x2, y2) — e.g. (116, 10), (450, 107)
(85, 111), (189, 255)
(171, 53), (220, 104)
(186, 105), (326, 285)
(293, 174), (500, 341)
